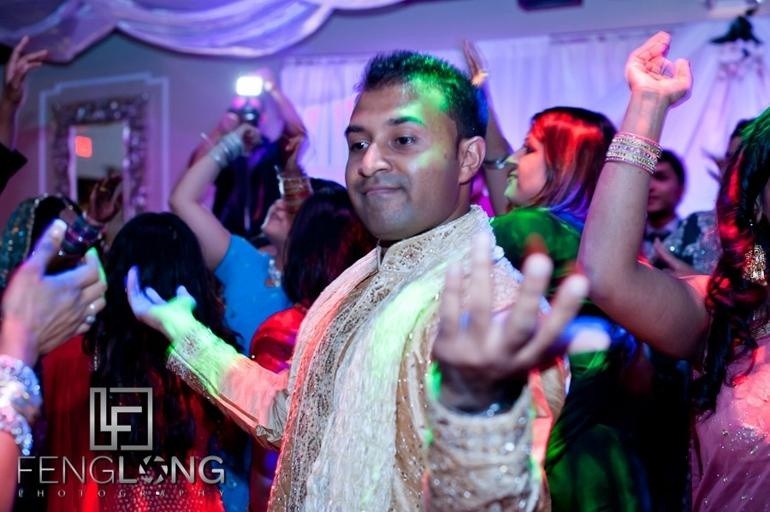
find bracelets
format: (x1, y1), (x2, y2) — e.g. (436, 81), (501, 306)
(604, 131), (665, 174)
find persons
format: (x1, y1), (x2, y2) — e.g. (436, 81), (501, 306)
(577, 31), (770, 512)
(125, 50), (589, 512)
(650, 119), (759, 379)
(458, 40), (635, 512)
(1, 32), (362, 512)
(644, 149), (687, 260)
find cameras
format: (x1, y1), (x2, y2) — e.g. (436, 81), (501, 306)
(226, 75), (266, 128)
